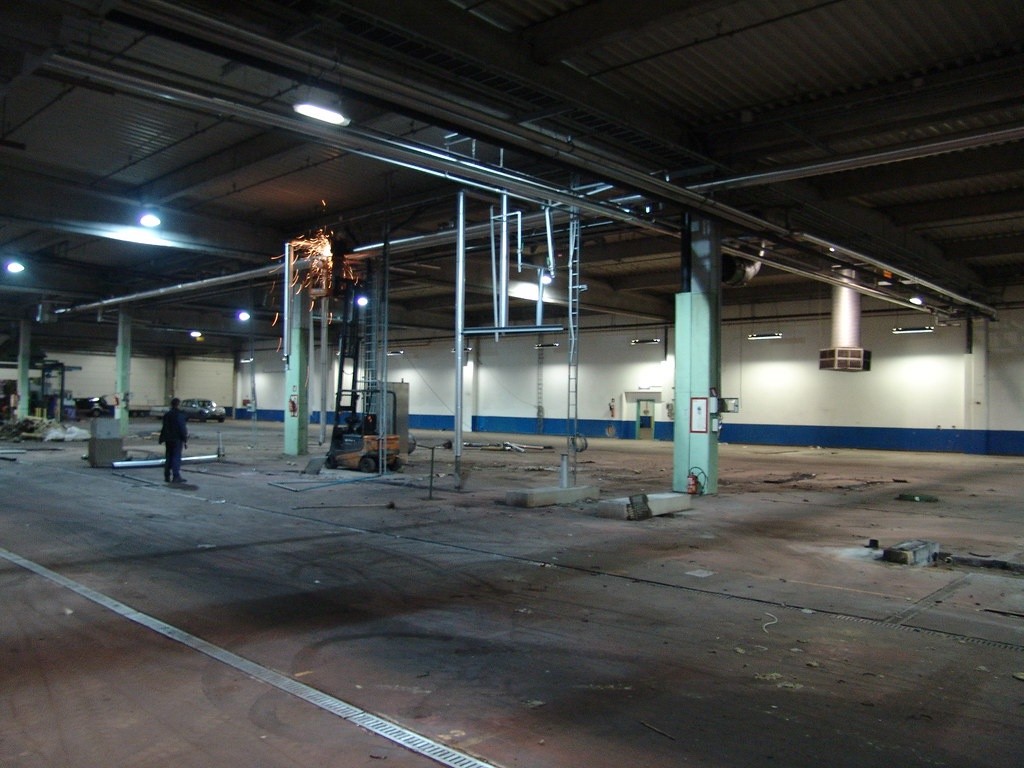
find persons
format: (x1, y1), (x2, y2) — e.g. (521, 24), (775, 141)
(158, 397), (190, 483)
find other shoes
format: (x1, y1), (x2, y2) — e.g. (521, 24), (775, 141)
(173, 477), (187, 484)
(165, 477), (171, 483)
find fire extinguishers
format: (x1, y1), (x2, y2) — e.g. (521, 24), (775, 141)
(687, 466), (707, 496)
(609, 398), (615, 417)
(289, 399), (295, 412)
(114, 395), (118, 405)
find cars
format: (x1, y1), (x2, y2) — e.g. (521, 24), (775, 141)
(178, 399), (226, 424)
(76, 397), (110, 418)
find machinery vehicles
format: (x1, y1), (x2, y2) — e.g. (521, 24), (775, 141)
(325, 245), (404, 474)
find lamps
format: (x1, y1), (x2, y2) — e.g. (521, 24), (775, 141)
(630, 316), (661, 345)
(746, 278), (783, 339)
(534, 334), (560, 349)
(892, 304), (935, 334)
(450, 336), (472, 353)
(387, 329), (404, 356)
